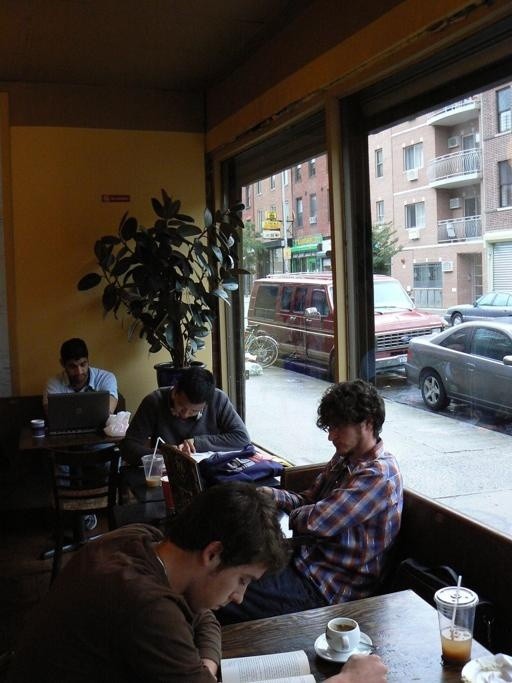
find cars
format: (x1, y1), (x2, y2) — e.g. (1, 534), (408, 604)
(403, 315), (512, 418)
(439, 289), (512, 328)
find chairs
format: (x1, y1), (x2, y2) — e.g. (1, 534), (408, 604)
(2, 387), (327, 585)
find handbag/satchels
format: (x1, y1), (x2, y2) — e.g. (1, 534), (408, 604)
(392, 557), (502, 655)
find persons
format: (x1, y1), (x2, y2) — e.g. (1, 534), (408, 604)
(1, 478), (397, 682)
(116, 366), (255, 470)
(39, 337), (119, 531)
(211, 379), (405, 628)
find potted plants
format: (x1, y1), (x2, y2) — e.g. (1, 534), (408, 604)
(74, 184), (251, 390)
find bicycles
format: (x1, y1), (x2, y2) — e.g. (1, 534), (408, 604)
(243, 324), (280, 369)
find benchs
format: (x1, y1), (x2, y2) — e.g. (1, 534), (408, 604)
(296, 483), (511, 666)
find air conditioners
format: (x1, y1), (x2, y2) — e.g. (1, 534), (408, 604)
(447, 134), (459, 149)
(448, 196), (462, 210)
(406, 226), (420, 239)
(405, 167), (418, 181)
(440, 258), (454, 272)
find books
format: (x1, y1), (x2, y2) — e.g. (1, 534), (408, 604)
(216, 646), (316, 682)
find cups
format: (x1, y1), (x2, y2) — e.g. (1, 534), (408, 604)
(31, 419), (45, 437)
(434, 586), (480, 669)
(141, 452), (164, 487)
(161, 476), (175, 510)
(326, 616), (361, 654)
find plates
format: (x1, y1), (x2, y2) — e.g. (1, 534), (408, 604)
(314, 631), (374, 663)
(461, 655), (511, 683)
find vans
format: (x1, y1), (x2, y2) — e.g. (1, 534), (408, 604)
(246, 268), (447, 391)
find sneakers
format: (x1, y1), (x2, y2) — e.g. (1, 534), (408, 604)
(79, 513), (98, 531)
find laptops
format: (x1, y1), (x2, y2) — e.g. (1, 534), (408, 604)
(45, 391), (110, 436)
(159, 443), (202, 515)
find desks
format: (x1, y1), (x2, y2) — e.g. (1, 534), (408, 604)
(219, 589), (507, 682)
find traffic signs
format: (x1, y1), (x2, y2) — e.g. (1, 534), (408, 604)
(262, 229), (282, 239)
(260, 218), (281, 229)
